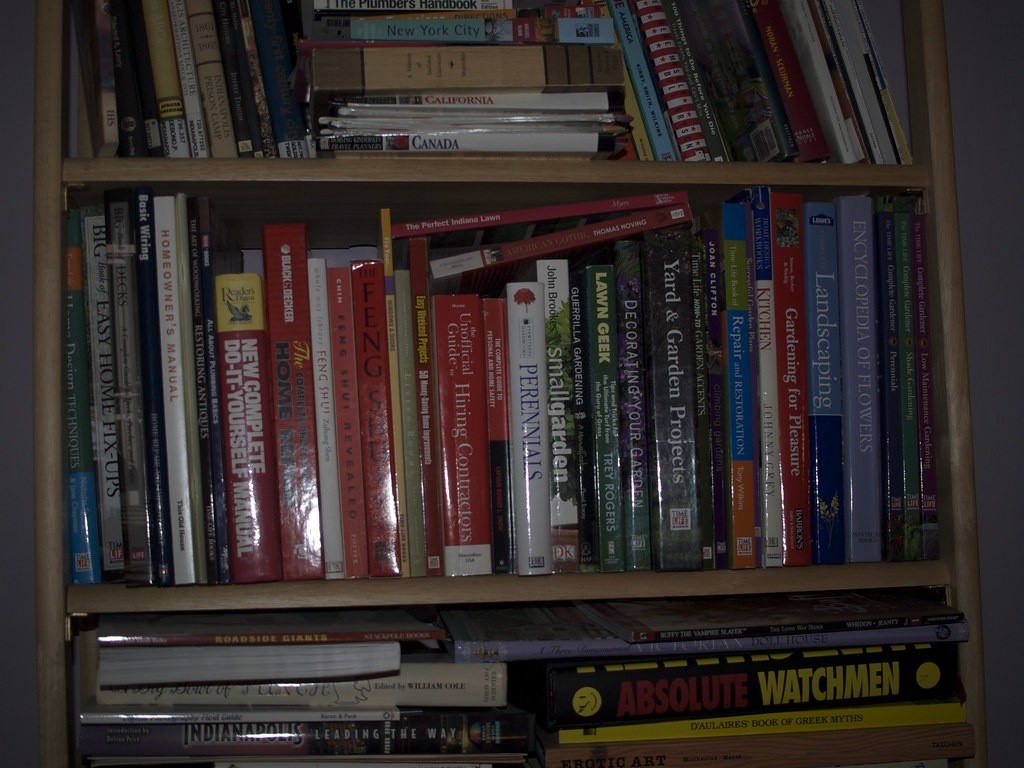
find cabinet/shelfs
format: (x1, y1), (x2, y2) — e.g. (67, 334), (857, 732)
(31, 0), (986, 767)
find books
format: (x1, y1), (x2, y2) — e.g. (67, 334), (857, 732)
(69, 608), (973, 760)
(63, 194), (941, 591)
(66, 0), (916, 166)
